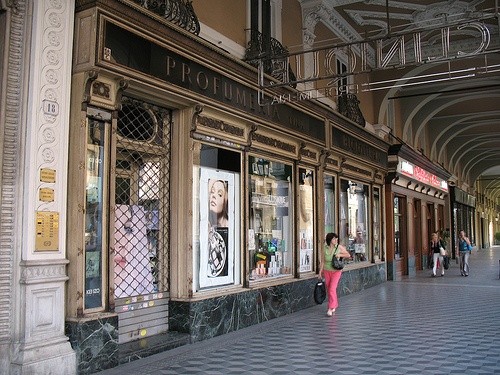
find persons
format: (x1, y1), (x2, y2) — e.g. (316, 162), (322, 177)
(318, 233), (350, 315)
(209, 180), (228, 228)
(431, 233), (445, 277)
(457, 230), (471, 276)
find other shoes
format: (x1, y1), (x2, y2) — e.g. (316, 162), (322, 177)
(465, 274), (468, 276)
(433, 275), (436, 277)
(441, 274), (444, 276)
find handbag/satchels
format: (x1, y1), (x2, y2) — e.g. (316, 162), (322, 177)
(444, 255), (450, 269)
(314, 278), (326, 304)
(332, 245), (344, 270)
(439, 240), (445, 255)
(467, 244), (472, 250)
